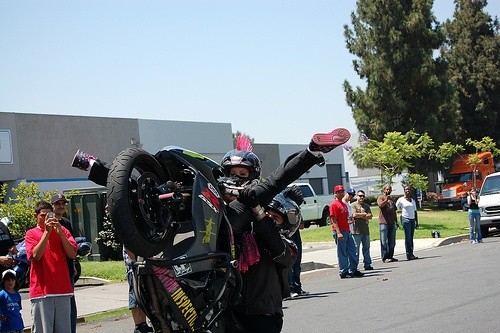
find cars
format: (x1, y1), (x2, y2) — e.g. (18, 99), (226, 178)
(478, 171), (500, 238)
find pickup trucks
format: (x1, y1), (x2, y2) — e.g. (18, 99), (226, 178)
(287, 182), (331, 229)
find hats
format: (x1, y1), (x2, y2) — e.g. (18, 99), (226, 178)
(50, 193), (68, 203)
(334, 185), (345, 193)
(347, 188), (355, 194)
(2, 269), (17, 281)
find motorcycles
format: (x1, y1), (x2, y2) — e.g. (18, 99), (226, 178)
(105, 144), (256, 333)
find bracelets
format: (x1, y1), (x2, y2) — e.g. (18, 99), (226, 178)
(254, 205), (265, 217)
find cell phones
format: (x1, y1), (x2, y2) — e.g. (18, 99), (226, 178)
(48, 212), (55, 226)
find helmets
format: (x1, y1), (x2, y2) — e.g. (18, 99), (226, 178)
(268, 194), (303, 240)
(219, 149), (261, 185)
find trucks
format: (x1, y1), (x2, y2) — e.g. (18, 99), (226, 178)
(437, 151), (495, 211)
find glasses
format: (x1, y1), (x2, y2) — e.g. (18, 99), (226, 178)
(347, 192), (356, 196)
(358, 195), (365, 198)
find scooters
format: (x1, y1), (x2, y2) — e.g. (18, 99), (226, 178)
(11, 233), (92, 291)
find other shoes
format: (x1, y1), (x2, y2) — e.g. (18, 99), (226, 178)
(339, 256), (418, 279)
(283, 291), (310, 300)
(134, 322), (154, 333)
(471, 239), (484, 245)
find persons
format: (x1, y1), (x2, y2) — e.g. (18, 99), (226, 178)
(467, 185), (484, 244)
(72, 128), (352, 259)
(328, 184), (364, 279)
(376, 185), (400, 263)
(0, 222), (19, 281)
(122, 243), (154, 333)
(395, 185), (419, 260)
(246, 192), (303, 301)
(288, 218), (310, 299)
(341, 188), (364, 277)
(350, 189), (374, 270)
(23, 192), (78, 333)
(0, 269), (25, 333)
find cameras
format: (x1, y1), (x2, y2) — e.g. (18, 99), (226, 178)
(469, 191), (476, 195)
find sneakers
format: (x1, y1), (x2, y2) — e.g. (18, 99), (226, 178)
(307, 128), (350, 153)
(71, 148), (95, 172)
